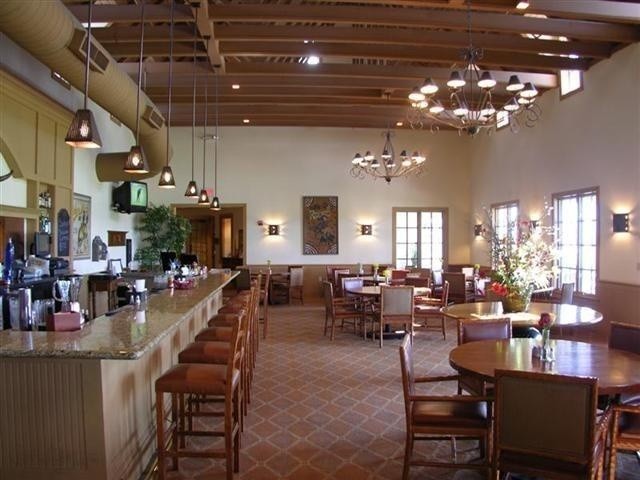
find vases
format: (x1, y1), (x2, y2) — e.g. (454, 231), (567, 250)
(538, 312), (555, 349)
(468, 198), (560, 297)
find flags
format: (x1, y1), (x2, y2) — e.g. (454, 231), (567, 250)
(501, 287), (533, 312)
(539, 329), (552, 362)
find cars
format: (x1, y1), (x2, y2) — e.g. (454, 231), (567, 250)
(9, 298), (19, 330)
(135, 280), (145, 292)
(31, 298), (56, 330)
(18, 287), (32, 331)
(15, 268), (24, 280)
(137, 310), (145, 324)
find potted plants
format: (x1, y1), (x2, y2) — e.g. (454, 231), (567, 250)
(71, 192), (91, 261)
(302, 196), (340, 256)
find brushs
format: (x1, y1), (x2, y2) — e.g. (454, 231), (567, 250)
(92, 236), (107, 262)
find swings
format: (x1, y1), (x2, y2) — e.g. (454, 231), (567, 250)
(50, 272), (89, 333)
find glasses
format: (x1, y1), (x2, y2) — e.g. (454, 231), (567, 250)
(474, 225), (481, 235)
(347, 89), (427, 184)
(361, 225), (372, 236)
(63, 0), (222, 212)
(613, 214), (629, 233)
(405, 0), (544, 140)
(269, 225), (277, 235)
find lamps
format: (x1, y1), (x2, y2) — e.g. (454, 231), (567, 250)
(154, 270), (272, 479)
(456, 318), (512, 401)
(606, 391), (640, 480)
(270, 265), (305, 304)
(608, 318), (640, 358)
(236, 264), (259, 292)
(486, 368), (614, 480)
(399, 333), (495, 480)
(318, 264), (495, 348)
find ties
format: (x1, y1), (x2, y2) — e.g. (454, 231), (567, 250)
(30, 231), (50, 256)
(112, 181), (148, 214)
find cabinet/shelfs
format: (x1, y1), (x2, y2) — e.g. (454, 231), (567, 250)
(168, 275), (173, 288)
(549, 347), (555, 361)
(2, 234), (15, 283)
(169, 261), (209, 278)
(130, 288), (150, 306)
(77, 211), (90, 254)
(38, 191), (53, 236)
(1, 270), (12, 285)
